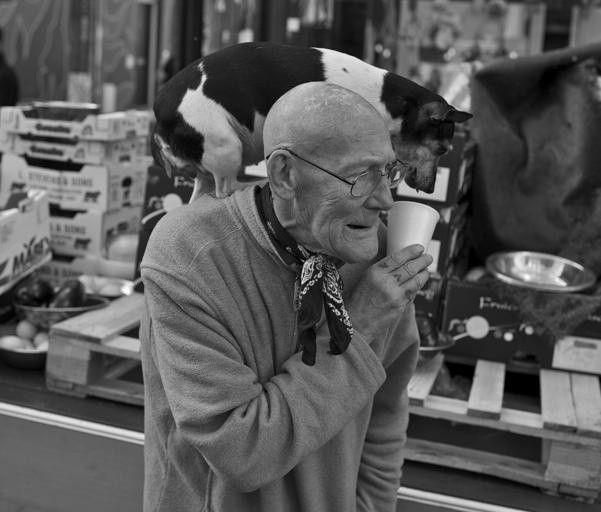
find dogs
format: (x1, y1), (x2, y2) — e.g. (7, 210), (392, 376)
(149, 41), (474, 204)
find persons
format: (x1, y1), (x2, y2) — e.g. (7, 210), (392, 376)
(372, 0), (529, 110)
(136, 80), (434, 512)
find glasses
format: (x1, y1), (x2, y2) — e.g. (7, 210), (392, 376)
(265, 145), (413, 199)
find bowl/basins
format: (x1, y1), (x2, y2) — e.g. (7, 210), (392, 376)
(32, 100), (98, 122)
(99, 277), (135, 301)
(0, 329), (49, 371)
(484, 249), (598, 295)
(415, 316), (456, 369)
(14, 295), (111, 333)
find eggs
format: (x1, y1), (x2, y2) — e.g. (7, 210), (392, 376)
(0, 318), (49, 353)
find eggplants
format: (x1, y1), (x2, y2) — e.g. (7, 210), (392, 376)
(18, 278), (87, 308)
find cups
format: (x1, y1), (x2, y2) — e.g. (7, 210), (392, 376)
(387, 200), (440, 259)
